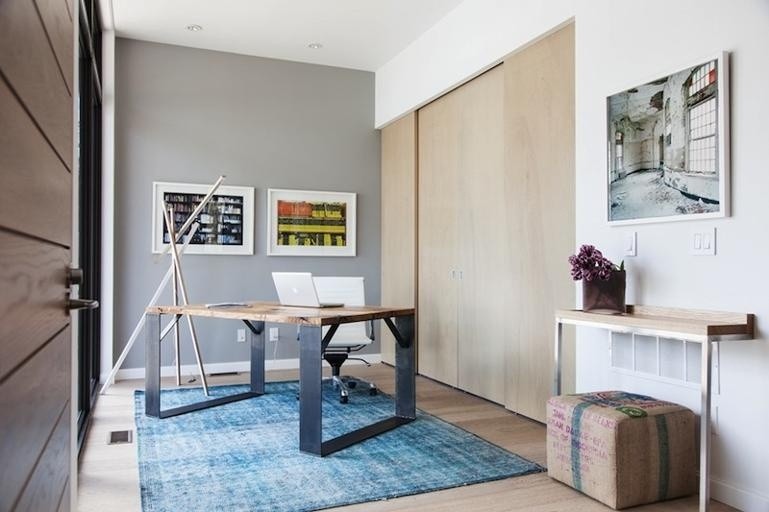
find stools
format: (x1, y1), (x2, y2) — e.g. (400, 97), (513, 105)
(549, 391), (699, 511)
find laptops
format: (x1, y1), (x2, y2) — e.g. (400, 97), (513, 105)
(271, 272), (344, 308)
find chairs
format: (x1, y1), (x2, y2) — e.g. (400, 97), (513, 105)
(297, 276), (378, 403)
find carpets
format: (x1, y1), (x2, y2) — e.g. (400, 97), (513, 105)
(135, 376), (548, 512)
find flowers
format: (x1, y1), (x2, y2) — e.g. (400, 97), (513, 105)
(569, 245), (611, 281)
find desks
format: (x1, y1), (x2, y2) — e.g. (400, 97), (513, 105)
(146, 301), (418, 456)
(555, 309), (756, 512)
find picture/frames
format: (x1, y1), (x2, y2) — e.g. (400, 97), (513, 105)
(152, 182), (255, 255)
(606, 49), (731, 225)
(268, 188), (357, 257)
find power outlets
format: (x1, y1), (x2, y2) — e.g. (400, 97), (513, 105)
(270, 328), (278, 341)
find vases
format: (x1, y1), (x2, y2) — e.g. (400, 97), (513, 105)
(583, 270), (626, 315)
